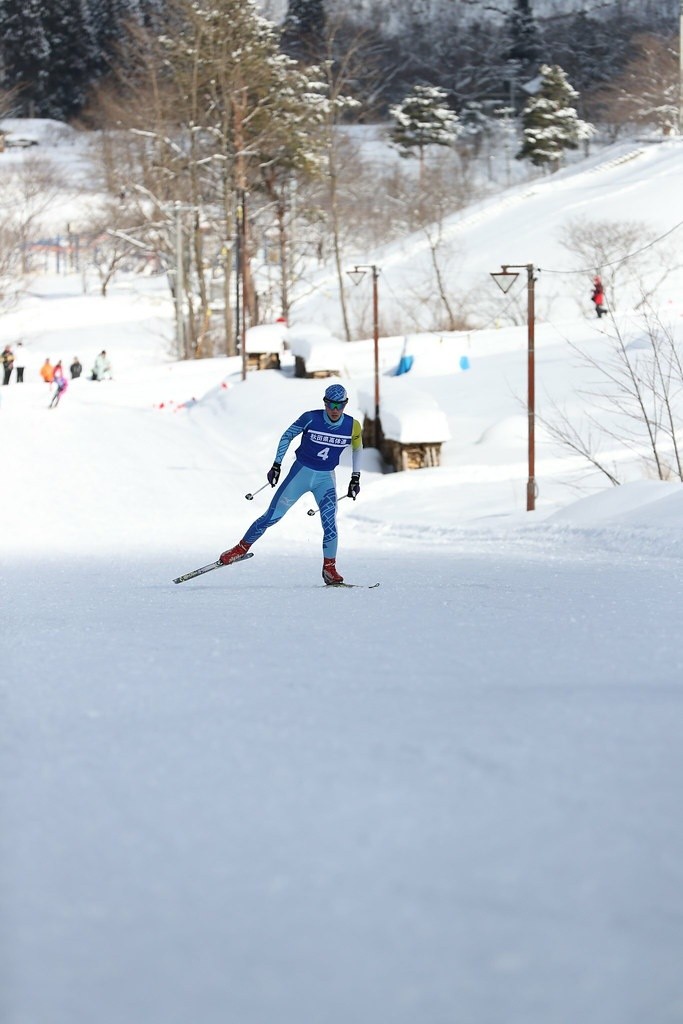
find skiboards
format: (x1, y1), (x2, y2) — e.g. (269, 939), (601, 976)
(172, 552), (381, 589)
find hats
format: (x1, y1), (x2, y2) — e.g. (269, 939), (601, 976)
(325, 385), (347, 401)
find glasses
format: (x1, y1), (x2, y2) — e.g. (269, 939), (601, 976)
(324, 398), (347, 410)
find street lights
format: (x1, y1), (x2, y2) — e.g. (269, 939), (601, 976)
(490, 264), (540, 512)
(347, 265), (381, 451)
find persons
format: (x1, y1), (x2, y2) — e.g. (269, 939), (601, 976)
(591, 276), (608, 318)
(40, 357), (82, 408)
(0, 342), (24, 385)
(220, 385), (363, 585)
(93, 351), (106, 380)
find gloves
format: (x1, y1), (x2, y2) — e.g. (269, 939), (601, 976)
(267, 462), (281, 485)
(347, 476), (360, 498)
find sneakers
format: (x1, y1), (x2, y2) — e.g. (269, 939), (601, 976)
(221, 540), (251, 564)
(322, 558), (343, 583)
